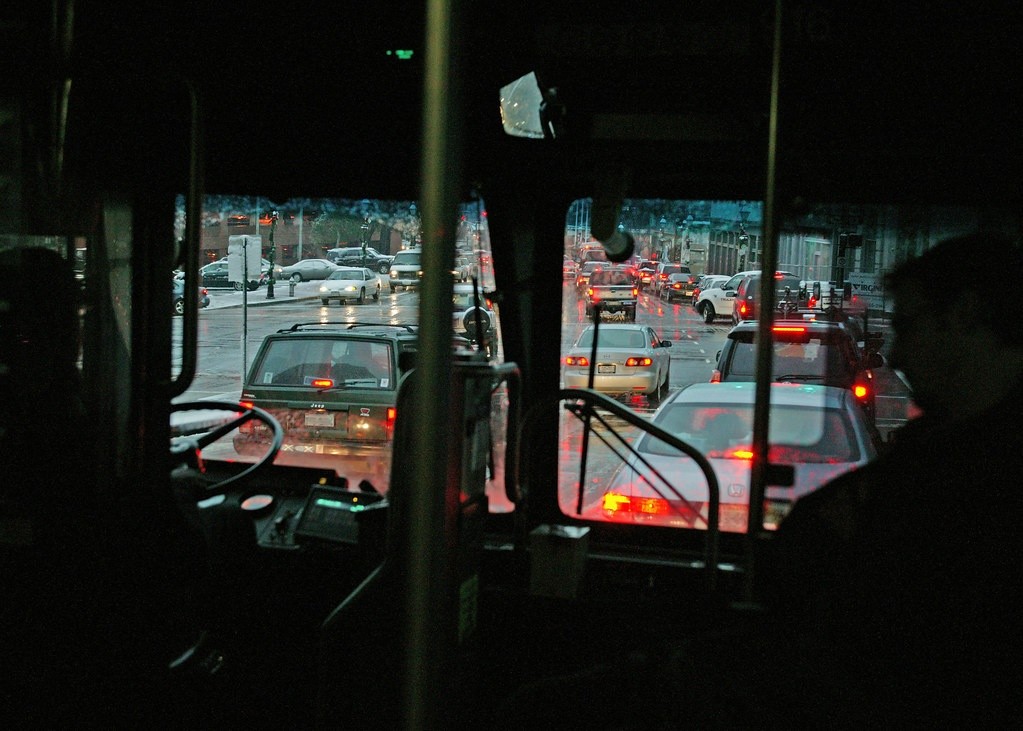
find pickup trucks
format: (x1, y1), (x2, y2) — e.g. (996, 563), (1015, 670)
(587, 270), (638, 322)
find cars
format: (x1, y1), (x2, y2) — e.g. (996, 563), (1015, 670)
(319, 267), (381, 305)
(659, 271), (695, 301)
(168, 279), (210, 315)
(282, 258), (358, 283)
(453, 249), (492, 283)
(173, 261), (264, 291)
(219, 254), (283, 285)
(563, 249), (659, 295)
(564, 322), (673, 403)
(602, 380), (900, 532)
(701, 279), (728, 290)
(696, 271), (760, 320)
(691, 275), (730, 306)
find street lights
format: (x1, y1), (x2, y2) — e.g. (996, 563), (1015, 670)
(660, 216), (666, 264)
(738, 203), (751, 272)
(265, 200), (277, 299)
(361, 199), (370, 267)
(410, 201), (417, 248)
(685, 215), (694, 264)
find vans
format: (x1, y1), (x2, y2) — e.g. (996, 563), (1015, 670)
(389, 249), (422, 293)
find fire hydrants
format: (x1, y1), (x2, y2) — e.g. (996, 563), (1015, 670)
(289, 276), (297, 297)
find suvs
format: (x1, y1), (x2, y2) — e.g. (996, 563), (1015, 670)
(709, 320), (876, 423)
(725, 272), (810, 328)
(328, 247), (395, 274)
(229, 317), (493, 478)
(650, 263), (690, 296)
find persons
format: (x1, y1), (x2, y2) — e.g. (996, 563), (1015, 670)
(776, 232), (1023, 552)
(0, 247), (256, 599)
